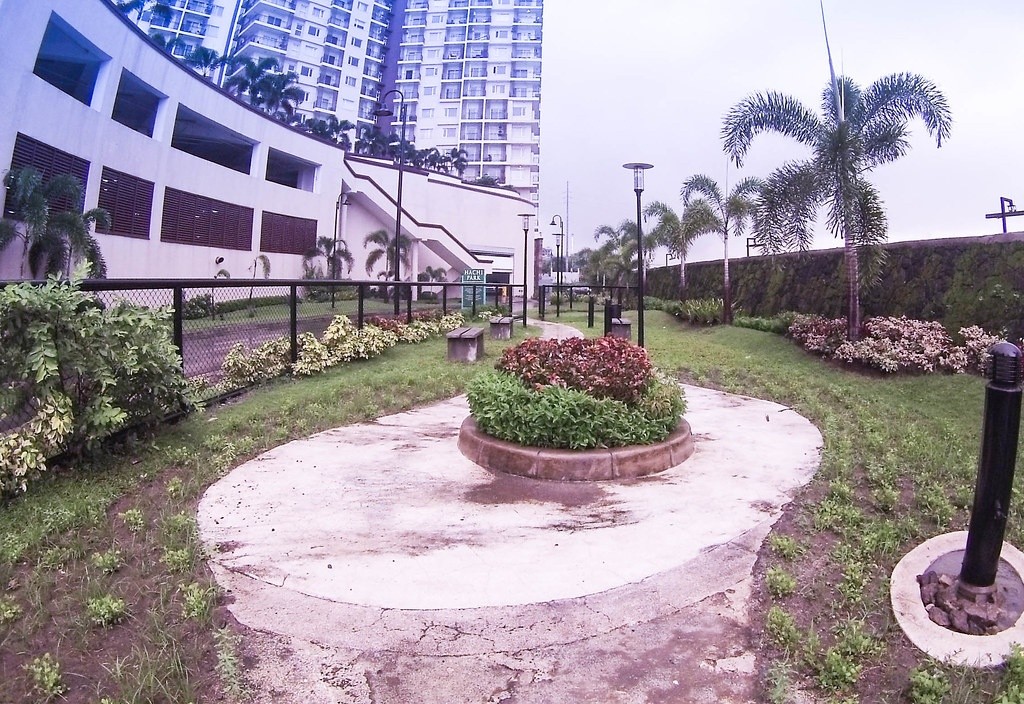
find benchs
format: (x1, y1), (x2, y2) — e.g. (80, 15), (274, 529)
(446, 327), (484, 363)
(489, 316), (513, 340)
(612, 317), (631, 342)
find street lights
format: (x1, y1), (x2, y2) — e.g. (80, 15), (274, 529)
(552, 214), (565, 317)
(665, 253), (676, 266)
(373, 88), (402, 315)
(517, 212), (536, 328)
(330, 193), (353, 308)
(624, 160), (655, 349)
(746, 236), (764, 256)
